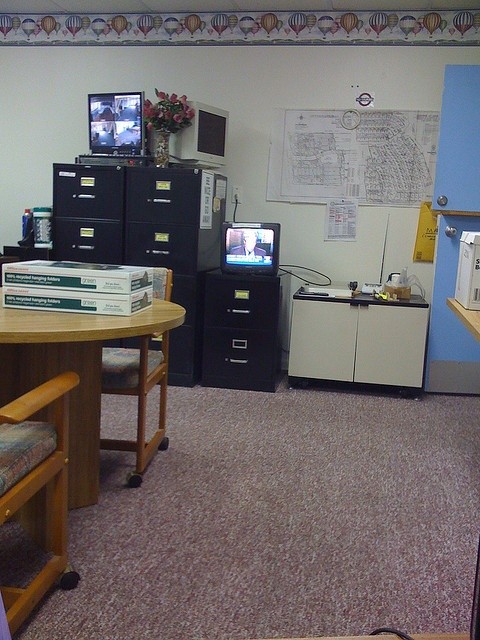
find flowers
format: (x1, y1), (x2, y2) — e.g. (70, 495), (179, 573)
(144, 88), (194, 132)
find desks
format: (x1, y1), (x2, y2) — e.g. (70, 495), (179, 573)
(0, 287), (187, 523)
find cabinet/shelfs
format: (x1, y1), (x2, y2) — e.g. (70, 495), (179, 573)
(52, 163), (123, 264)
(197, 269), (290, 394)
(4, 246), (50, 261)
(124, 168), (228, 391)
(287, 288), (429, 389)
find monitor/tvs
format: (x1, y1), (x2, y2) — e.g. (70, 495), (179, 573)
(83, 91), (146, 155)
(172, 101), (229, 167)
(218, 221), (281, 274)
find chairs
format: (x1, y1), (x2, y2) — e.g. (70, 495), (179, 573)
(99, 267), (172, 486)
(0, 370), (79, 640)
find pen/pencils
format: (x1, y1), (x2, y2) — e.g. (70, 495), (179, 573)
(299, 292), (329, 296)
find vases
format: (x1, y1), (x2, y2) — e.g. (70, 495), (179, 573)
(147, 127), (169, 168)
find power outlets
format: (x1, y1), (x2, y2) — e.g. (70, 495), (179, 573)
(232, 186), (243, 204)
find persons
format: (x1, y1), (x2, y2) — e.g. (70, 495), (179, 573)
(230, 231), (267, 256)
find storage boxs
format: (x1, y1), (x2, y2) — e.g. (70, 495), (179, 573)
(3, 259), (153, 294)
(4, 287), (152, 317)
(454, 232), (480, 311)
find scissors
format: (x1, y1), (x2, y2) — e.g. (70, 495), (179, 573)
(348, 281), (358, 291)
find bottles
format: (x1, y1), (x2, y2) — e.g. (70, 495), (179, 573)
(22, 208), (32, 240)
